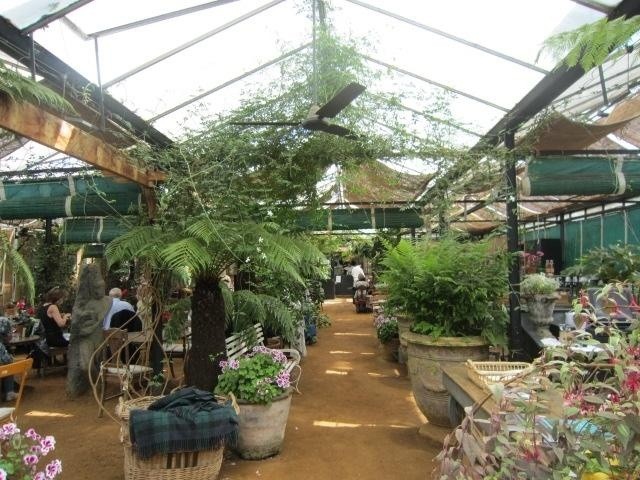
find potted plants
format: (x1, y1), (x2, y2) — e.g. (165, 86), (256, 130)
(518, 271), (563, 325)
(374, 230), (523, 429)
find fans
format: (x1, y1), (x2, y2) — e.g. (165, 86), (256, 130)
(228, 0), (366, 141)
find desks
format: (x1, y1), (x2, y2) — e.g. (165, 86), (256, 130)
(5, 335), (40, 361)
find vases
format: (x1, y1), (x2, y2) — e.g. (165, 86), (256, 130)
(214, 385), (294, 459)
(525, 266), (536, 274)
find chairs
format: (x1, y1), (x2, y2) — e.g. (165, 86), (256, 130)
(98, 328), (154, 419)
(0, 356), (33, 426)
(29, 317), (66, 377)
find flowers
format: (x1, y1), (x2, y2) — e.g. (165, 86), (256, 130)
(213, 344), (291, 407)
(0, 410), (62, 479)
(521, 250), (543, 266)
(15, 300), (35, 324)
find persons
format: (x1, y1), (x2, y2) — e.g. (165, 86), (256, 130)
(41, 287), (71, 347)
(0, 317), (19, 402)
(102, 287), (135, 330)
(351, 263), (369, 298)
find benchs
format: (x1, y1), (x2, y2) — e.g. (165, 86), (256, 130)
(225, 322), (301, 395)
